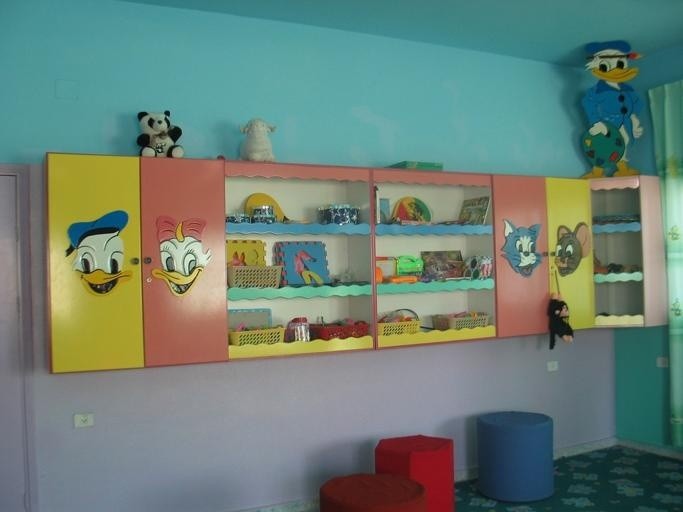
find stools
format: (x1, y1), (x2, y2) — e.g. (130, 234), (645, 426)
(320, 409), (556, 512)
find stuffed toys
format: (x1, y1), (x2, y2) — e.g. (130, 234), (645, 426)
(137, 110), (185, 158)
(549, 292), (574, 350)
(238, 118), (277, 162)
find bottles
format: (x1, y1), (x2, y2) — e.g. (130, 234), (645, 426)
(289, 318), (310, 342)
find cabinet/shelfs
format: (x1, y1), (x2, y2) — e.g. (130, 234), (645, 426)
(492, 170), (596, 340)
(370, 163), (494, 347)
(589, 173), (666, 330)
(225, 158), (375, 360)
(43, 152), (230, 375)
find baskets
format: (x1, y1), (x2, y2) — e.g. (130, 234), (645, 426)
(229, 327), (286, 346)
(228, 264), (284, 289)
(376, 320), (422, 336)
(308, 321), (370, 341)
(431, 313), (491, 330)
